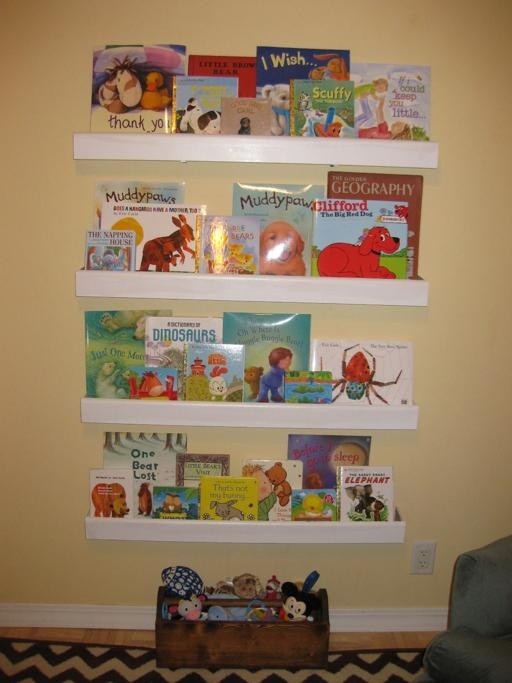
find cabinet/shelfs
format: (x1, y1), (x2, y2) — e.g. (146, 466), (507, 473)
(64, 134), (439, 545)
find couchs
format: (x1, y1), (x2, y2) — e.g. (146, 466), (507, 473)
(423, 535), (512, 681)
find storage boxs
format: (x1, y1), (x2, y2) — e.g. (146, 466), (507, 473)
(156, 586), (329, 668)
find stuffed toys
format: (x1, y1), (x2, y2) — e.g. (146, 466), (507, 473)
(159, 565), (321, 621)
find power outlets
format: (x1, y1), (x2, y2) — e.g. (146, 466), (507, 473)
(410, 542), (437, 573)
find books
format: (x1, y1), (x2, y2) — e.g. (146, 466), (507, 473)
(90, 431), (396, 523)
(90, 43), (433, 143)
(85, 172), (423, 281)
(84, 310), (414, 406)
